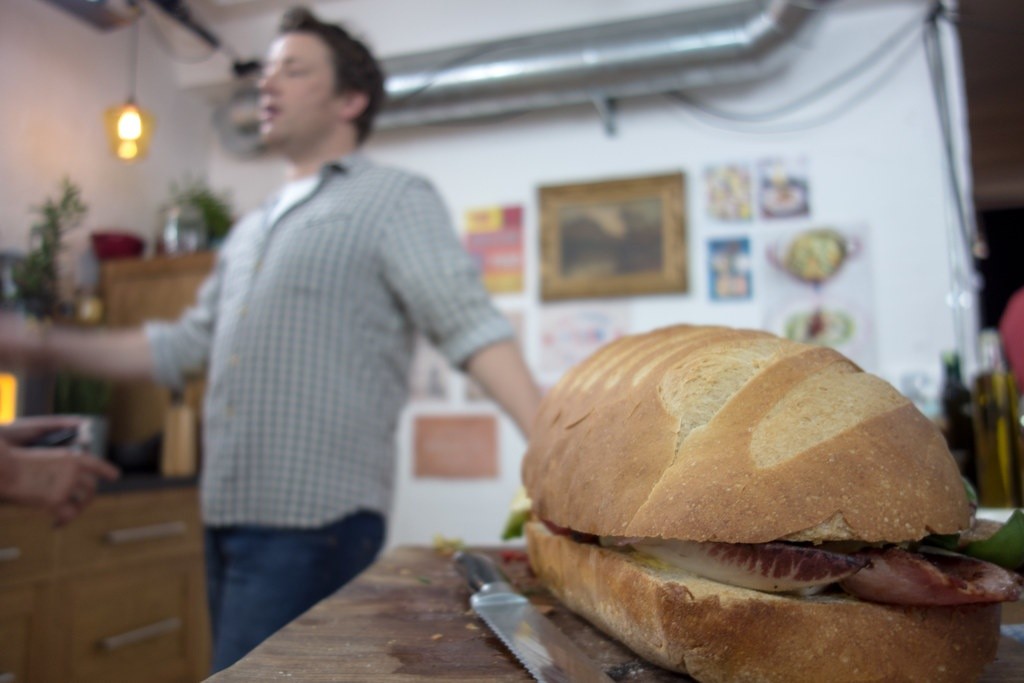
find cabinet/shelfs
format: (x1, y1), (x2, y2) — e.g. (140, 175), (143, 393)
(0, 483), (215, 683)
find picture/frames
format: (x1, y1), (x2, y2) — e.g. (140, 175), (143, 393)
(536, 170), (688, 301)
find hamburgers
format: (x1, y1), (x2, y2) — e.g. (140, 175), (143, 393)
(498, 324), (1024, 683)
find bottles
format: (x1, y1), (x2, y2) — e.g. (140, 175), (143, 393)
(157, 200), (208, 256)
(159, 389), (197, 478)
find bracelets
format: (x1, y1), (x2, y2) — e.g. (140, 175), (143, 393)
(37, 320), (51, 362)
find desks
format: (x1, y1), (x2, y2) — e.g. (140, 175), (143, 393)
(200, 544), (1024, 683)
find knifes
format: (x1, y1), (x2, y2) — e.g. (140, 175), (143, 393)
(452, 547), (613, 683)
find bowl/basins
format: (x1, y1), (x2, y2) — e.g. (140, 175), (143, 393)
(91, 232), (145, 263)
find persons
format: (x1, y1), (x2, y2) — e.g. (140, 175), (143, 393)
(0, 6), (547, 671)
